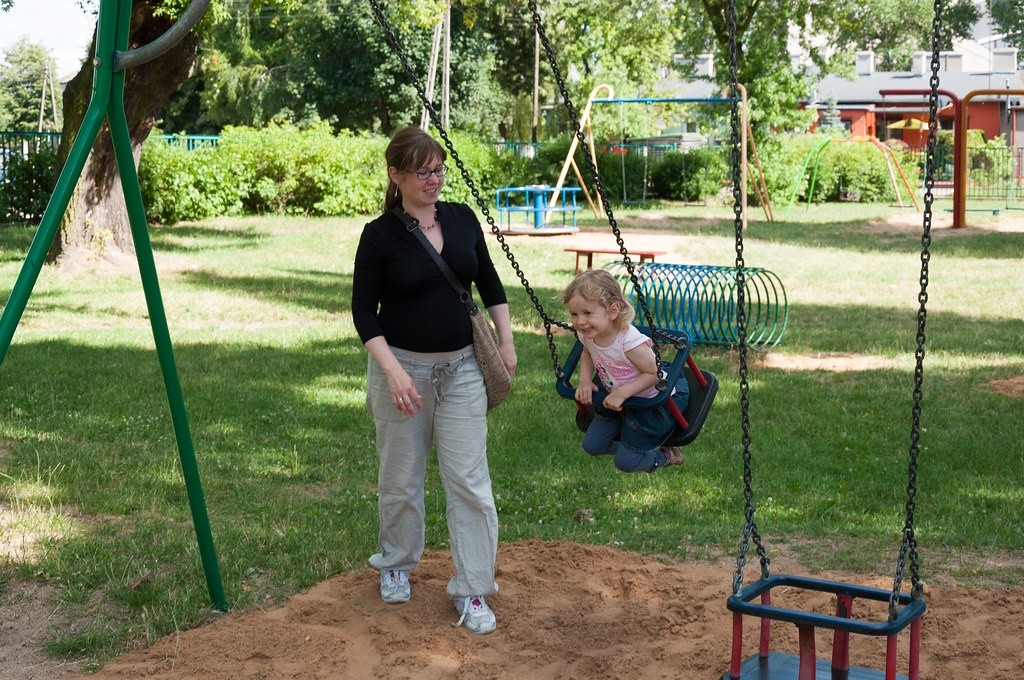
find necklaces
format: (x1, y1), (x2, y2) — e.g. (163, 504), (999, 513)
(401, 205), (438, 229)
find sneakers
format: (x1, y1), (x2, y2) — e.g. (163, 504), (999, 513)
(379, 568), (411, 603)
(450, 595), (496, 635)
(660, 444), (686, 466)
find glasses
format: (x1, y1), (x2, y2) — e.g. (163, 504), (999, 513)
(404, 163), (450, 181)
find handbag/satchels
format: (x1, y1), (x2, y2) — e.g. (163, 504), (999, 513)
(466, 303), (515, 413)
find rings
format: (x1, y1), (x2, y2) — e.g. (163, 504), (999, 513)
(397, 398), (402, 401)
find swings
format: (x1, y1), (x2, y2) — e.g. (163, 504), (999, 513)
(703, 5), (938, 679)
(371, 2), (719, 448)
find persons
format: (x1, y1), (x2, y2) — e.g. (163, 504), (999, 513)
(351, 123), (519, 634)
(549, 270), (685, 473)
